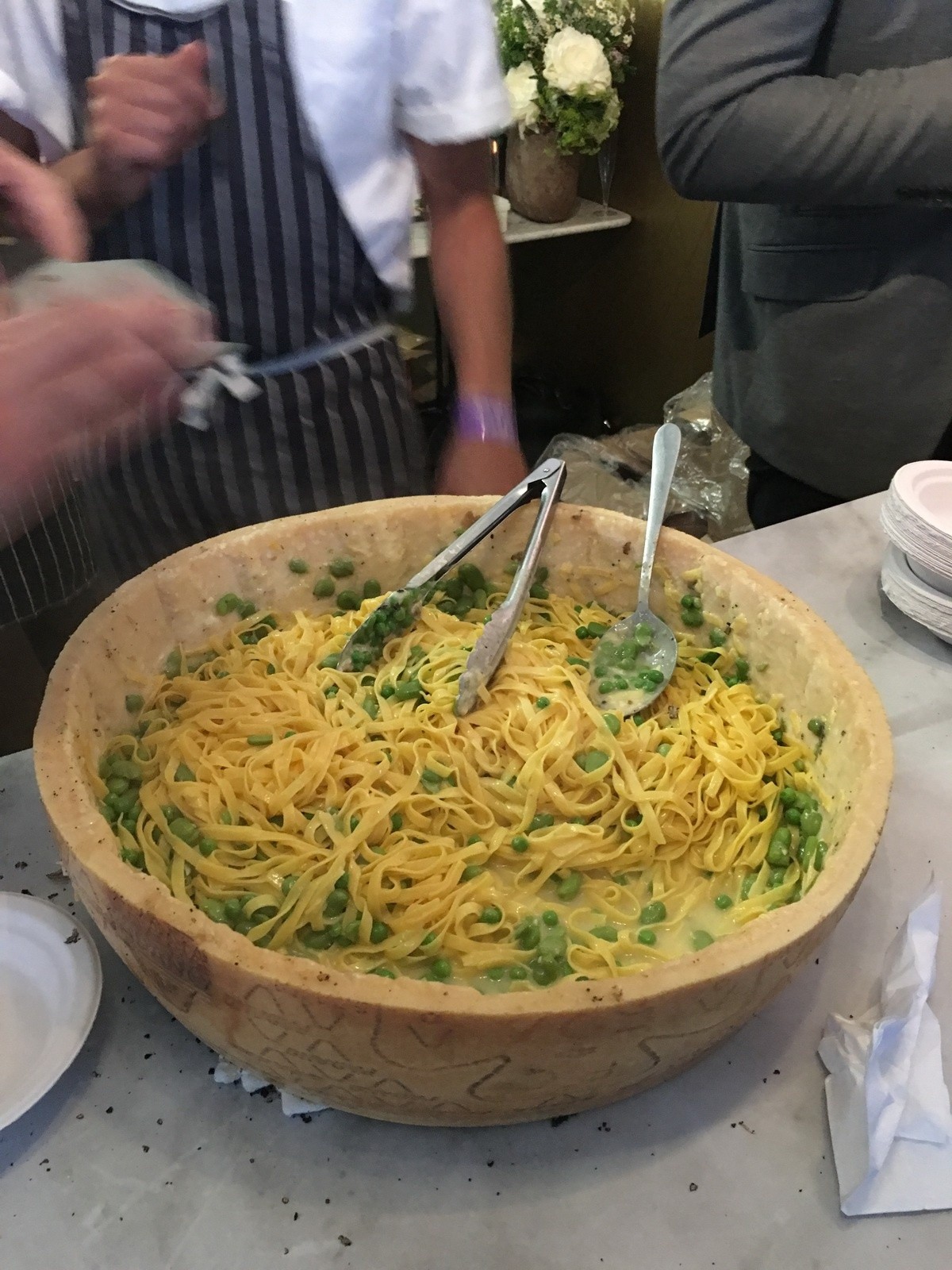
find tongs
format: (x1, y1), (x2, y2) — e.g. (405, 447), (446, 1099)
(333, 456), (570, 714)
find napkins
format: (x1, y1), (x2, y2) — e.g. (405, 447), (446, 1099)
(815, 888), (951, 1217)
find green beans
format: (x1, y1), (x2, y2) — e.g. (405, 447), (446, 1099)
(99, 558), (825, 983)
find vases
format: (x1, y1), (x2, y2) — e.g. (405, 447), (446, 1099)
(504, 130), (582, 226)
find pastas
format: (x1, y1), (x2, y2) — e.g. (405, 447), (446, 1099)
(85, 588), (834, 983)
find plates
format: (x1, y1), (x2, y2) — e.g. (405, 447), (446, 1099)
(1, 892), (102, 1131)
(879, 459), (951, 646)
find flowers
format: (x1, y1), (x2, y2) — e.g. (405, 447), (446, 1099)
(493, 0), (641, 156)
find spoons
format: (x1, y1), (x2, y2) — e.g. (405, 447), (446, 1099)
(587, 424), (682, 715)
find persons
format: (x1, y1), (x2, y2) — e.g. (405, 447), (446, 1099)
(656, 0), (952, 531)
(0, 0), (521, 757)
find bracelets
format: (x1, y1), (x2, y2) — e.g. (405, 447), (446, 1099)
(455, 395), (519, 443)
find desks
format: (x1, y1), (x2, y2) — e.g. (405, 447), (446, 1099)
(413, 193), (632, 430)
(0, 495), (952, 1270)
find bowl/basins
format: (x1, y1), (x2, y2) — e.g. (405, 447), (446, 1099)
(31, 495), (894, 1127)
